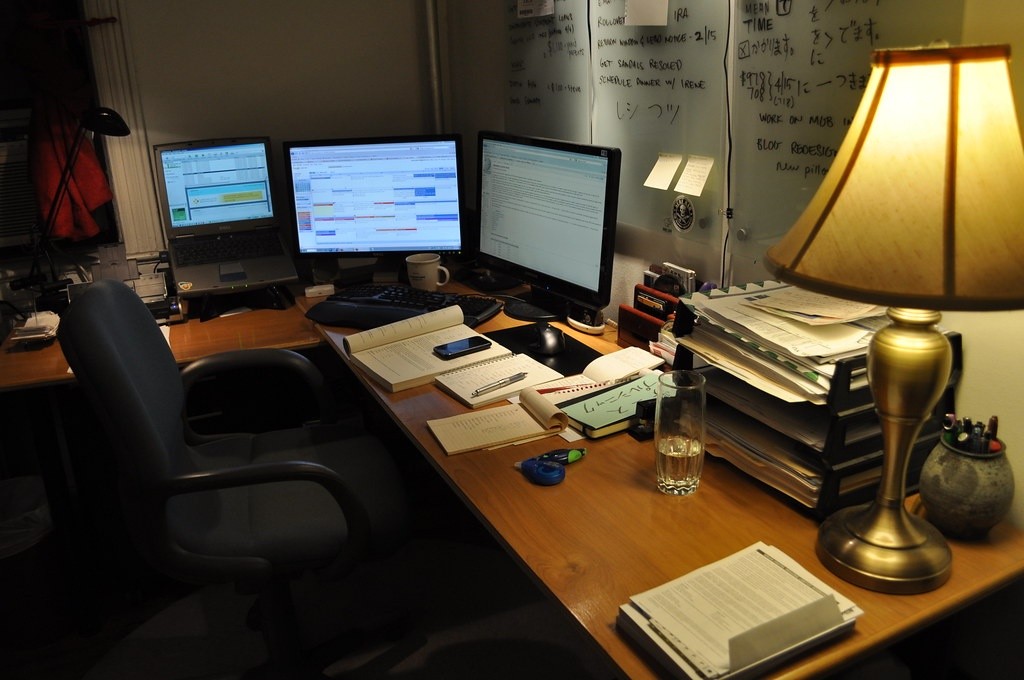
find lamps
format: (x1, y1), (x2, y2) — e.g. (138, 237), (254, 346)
(761, 42), (1024, 593)
(7, 107), (136, 351)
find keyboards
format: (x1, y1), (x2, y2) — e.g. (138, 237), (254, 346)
(305, 284), (504, 328)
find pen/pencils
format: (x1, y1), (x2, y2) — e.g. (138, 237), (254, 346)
(942, 412), (1001, 454)
(471, 371), (529, 397)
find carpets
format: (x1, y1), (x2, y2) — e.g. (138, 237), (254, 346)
(0, 534), (930, 680)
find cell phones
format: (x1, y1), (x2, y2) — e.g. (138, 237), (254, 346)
(433, 336), (492, 360)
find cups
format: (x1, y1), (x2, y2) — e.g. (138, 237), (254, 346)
(654, 371), (706, 496)
(919, 434), (1014, 536)
(406, 254), (449, 291)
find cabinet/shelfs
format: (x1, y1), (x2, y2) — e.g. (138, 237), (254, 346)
(670, 277), (963, 529)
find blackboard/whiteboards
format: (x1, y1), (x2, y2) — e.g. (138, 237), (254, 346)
(433, 1), (964, 289)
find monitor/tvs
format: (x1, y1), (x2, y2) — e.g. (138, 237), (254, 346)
(283, 133), (467, 289)
(474, 130), (622, 320)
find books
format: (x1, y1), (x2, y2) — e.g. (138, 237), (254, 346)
(425, 388), (568, 457)
(509, 346), (676, 440)
(435, 353), (564, 410)
(343, 304), (514, 392)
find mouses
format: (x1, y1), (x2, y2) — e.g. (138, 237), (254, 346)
(529, 323), (565, 354)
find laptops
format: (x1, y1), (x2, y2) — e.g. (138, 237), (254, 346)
(154, 137), (298, 298)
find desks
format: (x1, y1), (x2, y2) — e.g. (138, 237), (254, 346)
(0, 274), (1020, 680)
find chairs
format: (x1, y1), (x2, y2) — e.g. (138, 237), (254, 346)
(56, 280), (420, 680)
